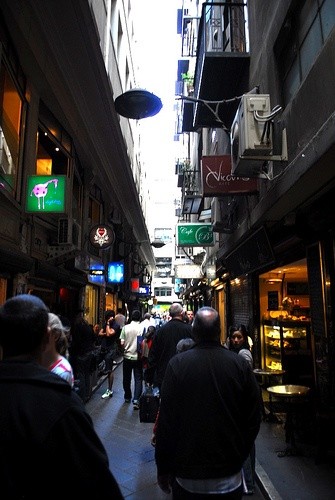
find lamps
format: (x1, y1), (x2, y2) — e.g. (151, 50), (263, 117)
(120, 240), (165, 249)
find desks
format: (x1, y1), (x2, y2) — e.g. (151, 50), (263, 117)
(266, 384), (311, 458)
(253, 367), (288, 423)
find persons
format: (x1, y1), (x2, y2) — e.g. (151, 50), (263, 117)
(48, 302), (262, 500)
(0, 293), (125, 500)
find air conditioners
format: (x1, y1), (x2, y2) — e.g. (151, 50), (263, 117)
(211, 196), (230, 229)
(56, 217), (82, 252)
(229, 93), (274, 177)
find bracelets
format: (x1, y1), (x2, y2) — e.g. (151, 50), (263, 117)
(107, 321), (110, 325)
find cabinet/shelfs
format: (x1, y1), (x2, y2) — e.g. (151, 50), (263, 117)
(260, 313), (315, 402)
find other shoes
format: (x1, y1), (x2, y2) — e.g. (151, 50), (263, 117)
(125, 399), (131, 402)
(132, 404), (139, 410)
(101, 388), (113, 399)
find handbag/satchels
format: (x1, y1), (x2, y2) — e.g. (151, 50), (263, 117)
(138, 388), (159, 423)
